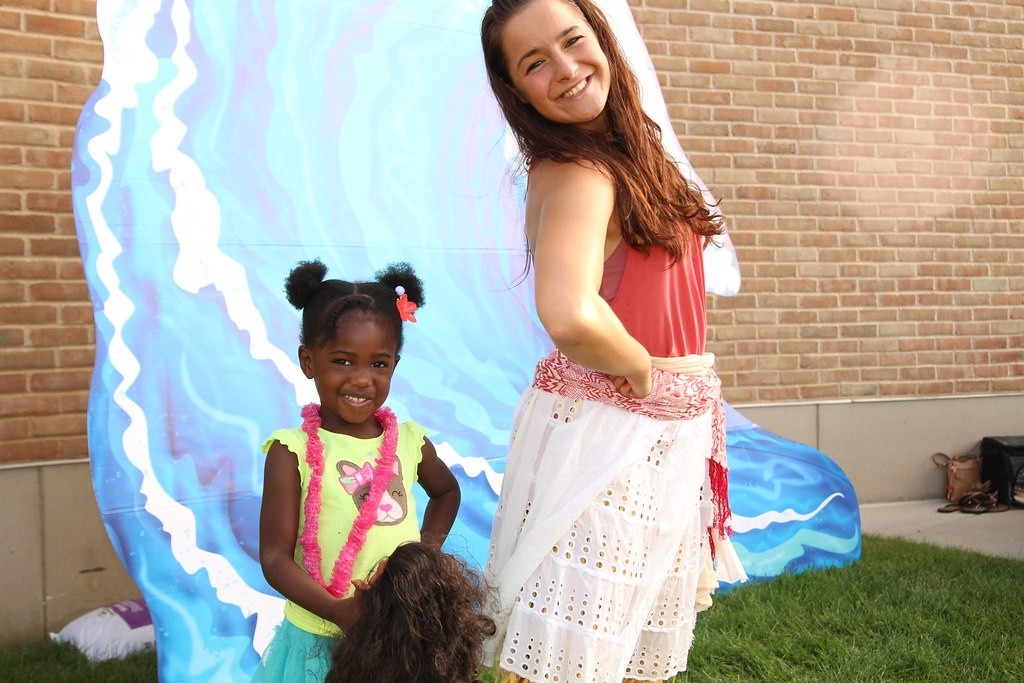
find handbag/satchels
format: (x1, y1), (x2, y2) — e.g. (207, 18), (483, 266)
(933, 453), (981, 501)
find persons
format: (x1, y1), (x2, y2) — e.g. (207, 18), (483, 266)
(474, 0), (749, 683)
(251, 258), (497, 683)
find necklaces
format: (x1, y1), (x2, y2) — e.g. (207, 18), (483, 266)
(299, 402), (399, 600)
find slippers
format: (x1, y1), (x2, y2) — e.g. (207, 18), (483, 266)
(937, 492), (1009, 513)
